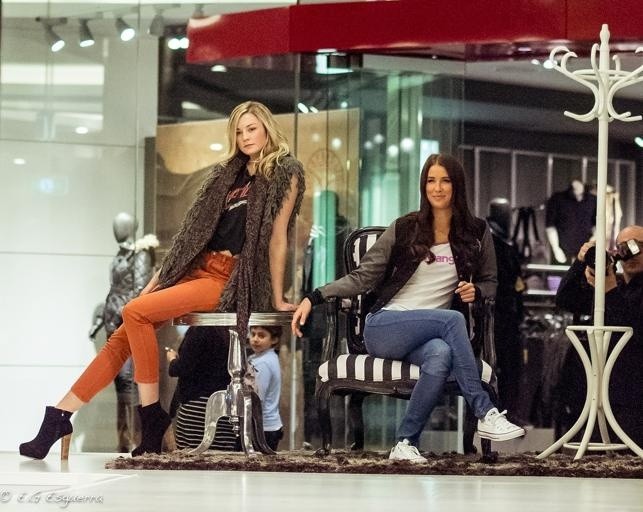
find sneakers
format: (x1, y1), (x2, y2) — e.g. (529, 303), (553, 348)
(477, 406), (525, 442)
(389, 438), (428, 465)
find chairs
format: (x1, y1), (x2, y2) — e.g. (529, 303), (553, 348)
(317, 225), (499, 462)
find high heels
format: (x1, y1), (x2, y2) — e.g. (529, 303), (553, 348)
(19, 405), (73, 460)
(131, 400), (177, 458)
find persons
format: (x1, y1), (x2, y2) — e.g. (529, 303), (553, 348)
(554, 225), (643, 455)
(165, 325), (242, 452)
(544, 179), (600, 265)
(290, 156), (523, 468)
(19, 100), (305, 459)
(247, 325), (285, 453)
(482, 197), (529, 427)
(90, 211), (153, 453)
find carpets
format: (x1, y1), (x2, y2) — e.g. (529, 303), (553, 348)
(106, 446), (643, 480)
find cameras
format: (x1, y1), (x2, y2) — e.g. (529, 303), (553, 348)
(583, 238), (641, 276)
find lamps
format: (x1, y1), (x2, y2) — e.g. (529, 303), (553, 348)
(76, 23), (96, 49)
(114, 19), (136, 44)
(43, 24), (66, 53)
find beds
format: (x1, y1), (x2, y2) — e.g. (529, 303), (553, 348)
(171, 310), (300, 463)
